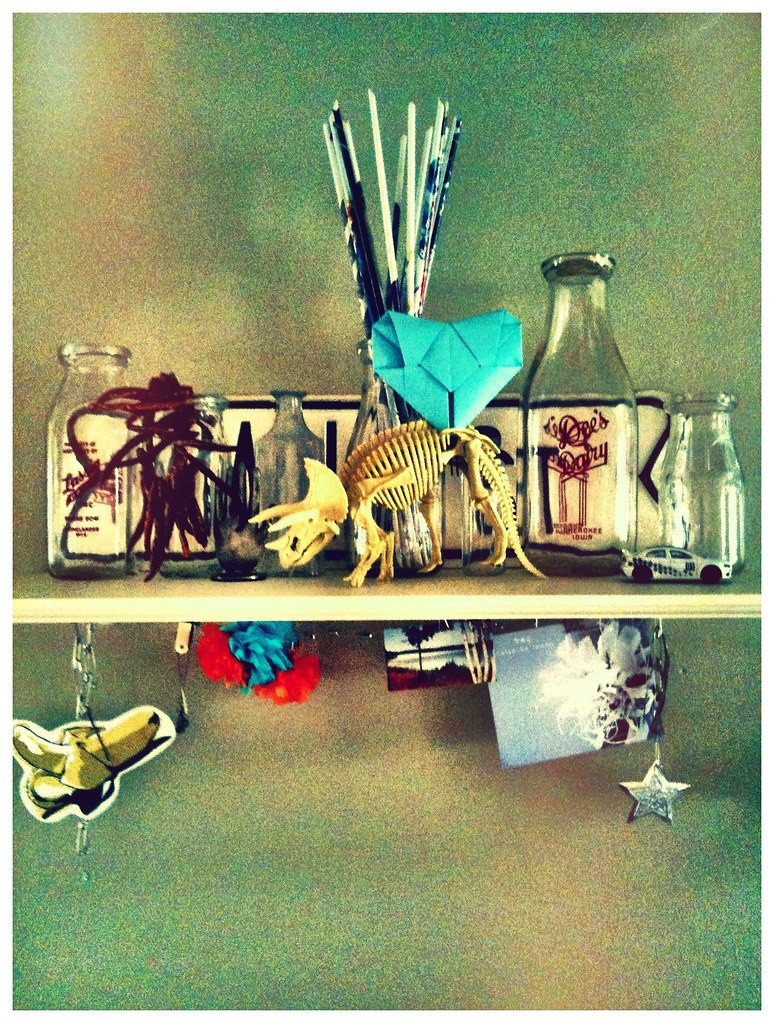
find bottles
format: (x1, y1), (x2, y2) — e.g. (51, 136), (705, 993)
(524, 252), (640, 575)
(345, 339), (444, 578)
(254, 389), (326, 577)
(657, 393), (747, 573)
(150, 395), (229, 577)
(45, 340), (141, 578)
